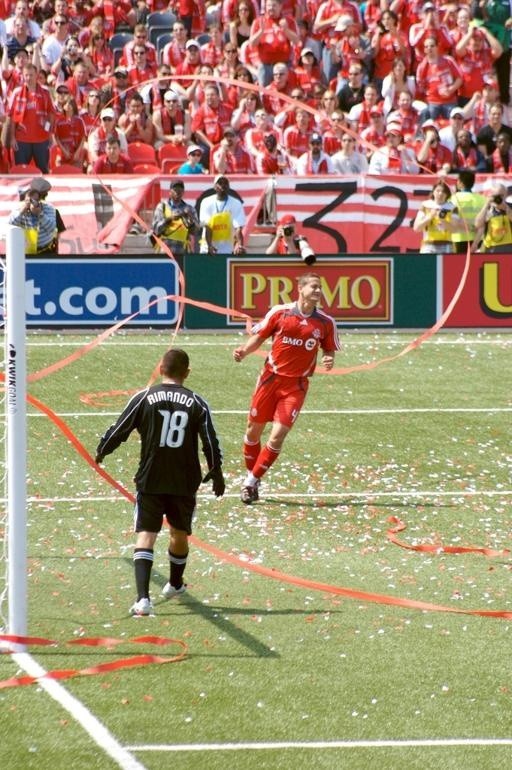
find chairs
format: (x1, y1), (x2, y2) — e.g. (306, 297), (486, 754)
(112, 13), (225, 70)
(9, 142), (220, 175)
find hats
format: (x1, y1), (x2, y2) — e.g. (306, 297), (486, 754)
(54, 3), (486, 156)
(170, 180), (184, 189)
(214, 175), (223, 183)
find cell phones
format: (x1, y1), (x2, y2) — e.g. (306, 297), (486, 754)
(439, 208), (446, 218)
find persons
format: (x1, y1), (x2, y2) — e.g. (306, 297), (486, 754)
(89, 347), (225, 618)
(414, 179), (463, 252)
(10, 177), (67, 255)
(152, 179), (200, 253)
(233, 274), (337, 502)
(448, 170), (487, 252)
(199, 175), (247, 255)
(0, 1), (512, 175)
(475, 183), (512, 253)
(266, 215), (307, 256)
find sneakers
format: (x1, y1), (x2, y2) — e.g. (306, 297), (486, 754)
(129, 598), (151, 615)
(245, 470), (258, 500)
(162, 582), (186, 599)
(240, 479), (253, 502)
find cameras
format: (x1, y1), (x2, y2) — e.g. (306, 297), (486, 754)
(493, 195), (502, 204)
(294, 236), (315, 265)
(179, 212), (192, 228)
(283, 226), (293, 236)
(29, 198), (40, 207)
(67, 48), (77, 53)
